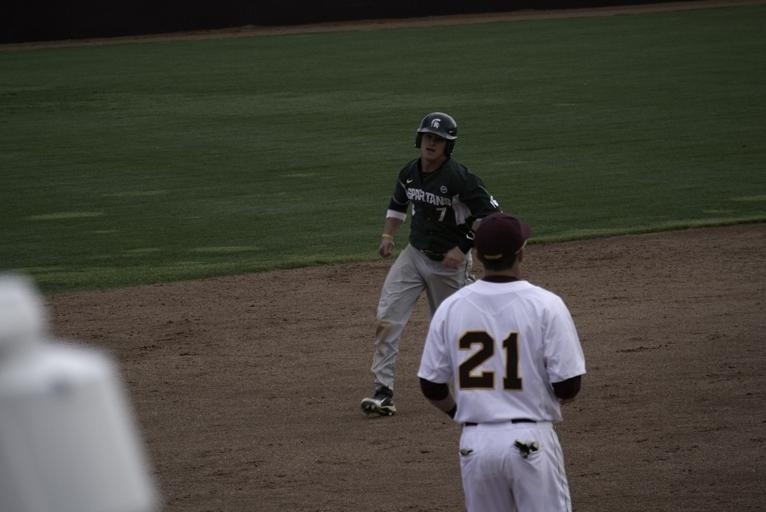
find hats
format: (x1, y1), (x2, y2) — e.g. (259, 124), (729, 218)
(473, 213), (529, 261)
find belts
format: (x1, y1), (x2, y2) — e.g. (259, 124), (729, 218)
(461, 418), (538, 426)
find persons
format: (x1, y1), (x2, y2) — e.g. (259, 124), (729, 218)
(0, 271), (162, 511)
(359, 111), (503, 417)
(416, 213), (586, 512)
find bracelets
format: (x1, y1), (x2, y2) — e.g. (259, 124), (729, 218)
(447, 404), (457, 418)
(382, 233), (394, 239)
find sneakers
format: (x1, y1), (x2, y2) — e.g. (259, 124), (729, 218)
(360, 394), (397, 417)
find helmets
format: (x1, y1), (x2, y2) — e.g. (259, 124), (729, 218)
(414, 110), (459, 157)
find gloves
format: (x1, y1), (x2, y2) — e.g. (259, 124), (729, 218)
(514, 439), (539, 459)
